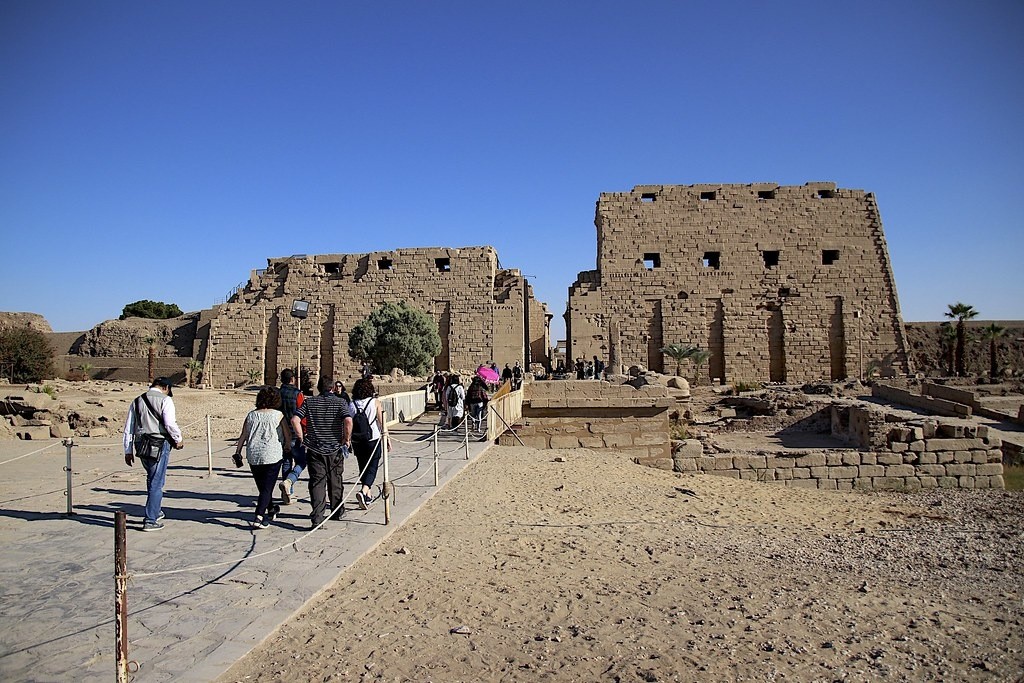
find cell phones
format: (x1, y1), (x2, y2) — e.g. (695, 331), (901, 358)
(233, 454), (243, 467)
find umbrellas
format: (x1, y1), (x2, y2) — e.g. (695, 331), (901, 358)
(478, 365), (499, 381)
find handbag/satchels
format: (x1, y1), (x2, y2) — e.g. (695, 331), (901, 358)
(434, 389), (438, 393)
(135, 433), (165, 462)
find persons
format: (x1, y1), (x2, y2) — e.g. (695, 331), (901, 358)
(443, 372), (449, 384)
(433, 369), (444, 409)
(290, 375), (353, 529)
(334, 381), (350, 405)
(557, 362), (564, 370)
(349, 379), (392, 509)
(593, 355), (601, 380)
(503, 363), (512, 383)
(513, 362), (522, 391)
(278, 368), (307, 504)
(575, 358), (584, 380)
(123, 377), (183, 531)
(442, 374), (465, 433)
(490, 363), (499, 393)
(585, 361), (594, 379)
(236, 385), (292, 528)
(467, 376), (489, 432)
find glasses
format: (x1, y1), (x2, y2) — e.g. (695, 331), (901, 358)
(335, 385), (342, 387)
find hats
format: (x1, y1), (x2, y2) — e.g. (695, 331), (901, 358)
(155, 377), (173, 397)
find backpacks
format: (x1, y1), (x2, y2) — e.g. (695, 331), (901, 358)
(447, 384), (460, 407)
(352, 397), (376, 442)
(469, 383), (482, 403)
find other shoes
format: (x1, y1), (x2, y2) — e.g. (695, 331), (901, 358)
(356, 491), (368, 510)
(312, 522), (327, 530)
(366, 494), (379, 504)
(291, 495), (297, 502)
(279, 480), (292, 504)
(332, 510), (347, 519)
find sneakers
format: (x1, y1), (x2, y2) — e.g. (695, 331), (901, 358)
(268, 504), (280, 521)
(253, 517), (270, 528)
(143, 522), (165, 531)
(156, 510), (165, 521)
